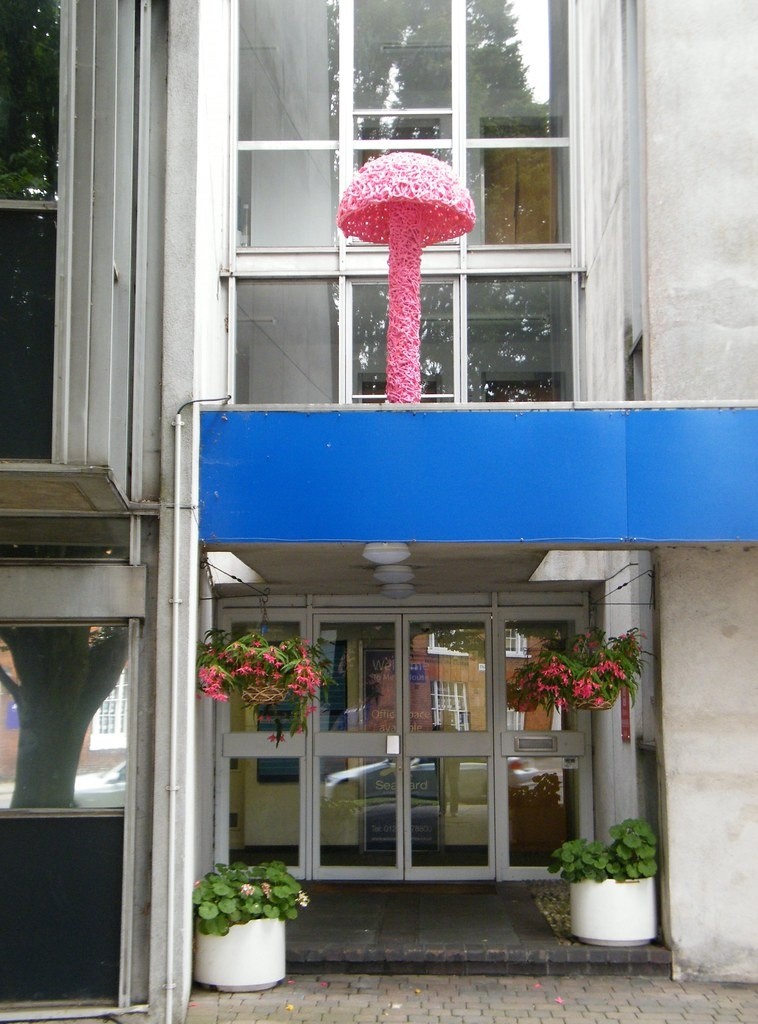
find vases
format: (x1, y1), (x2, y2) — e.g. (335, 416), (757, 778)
(574, 681), (620, 711)
(238, 675), (288, 704)
(194, 914), (288, 996)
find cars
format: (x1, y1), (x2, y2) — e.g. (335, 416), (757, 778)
(73, 760), (127, 808)
(327, 756), (542, 802)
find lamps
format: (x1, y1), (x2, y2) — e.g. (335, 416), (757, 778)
(360, 541), (419, 601)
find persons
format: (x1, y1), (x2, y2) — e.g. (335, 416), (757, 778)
(432, 710), (460, 816)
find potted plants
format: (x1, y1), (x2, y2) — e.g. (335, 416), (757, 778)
(507, 771), (564, 855)
(552, 817), (666, 949)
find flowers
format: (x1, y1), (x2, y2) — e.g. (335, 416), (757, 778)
(194, 617), (335, 743)
(506, 612), (647, 711)
(195, 855), (314, 933)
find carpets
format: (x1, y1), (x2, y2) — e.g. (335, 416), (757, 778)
(523, 878), (665, 949)
